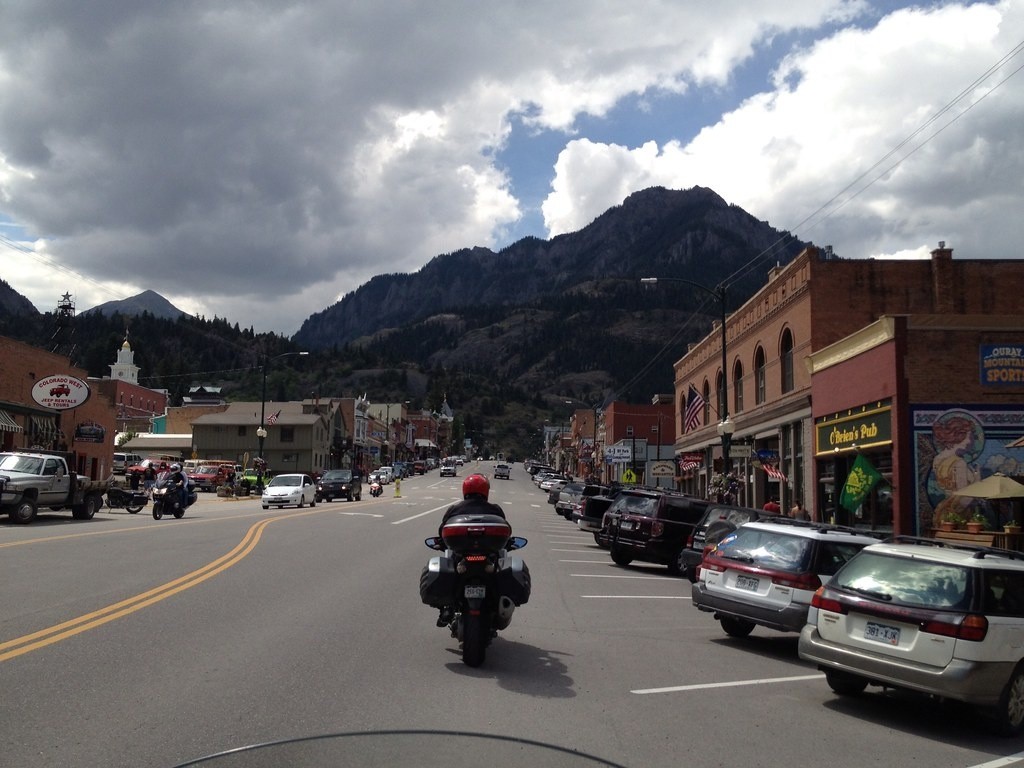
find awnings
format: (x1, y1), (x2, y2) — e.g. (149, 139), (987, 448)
(415, 439), (436, 447)
(28, 414), (64, 440)
(0, 408), (24, 433)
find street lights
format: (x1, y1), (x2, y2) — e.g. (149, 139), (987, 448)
(565, 401), (598, 486)
(641, 276), (737, 507)
(385, 401), (410, 467)
(254, 352), (309, 495)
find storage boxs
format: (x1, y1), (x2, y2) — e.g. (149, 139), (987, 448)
(441, 515), (512, 555)
(419, 557), (454, 605)
(497, 556), (531, 608)
(187, 480), (195, 491)
(188, 493), (197, 504)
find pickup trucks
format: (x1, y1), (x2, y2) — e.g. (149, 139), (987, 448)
(187, 465), (235, 493)
(0, 451), (109, 525)
(126, 459), (179, 481)
(493, 464), (512, 480)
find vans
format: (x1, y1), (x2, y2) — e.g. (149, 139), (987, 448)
(220, 463), (244, 484)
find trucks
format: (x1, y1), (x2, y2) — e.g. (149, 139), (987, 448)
(496, 452), (505, 461)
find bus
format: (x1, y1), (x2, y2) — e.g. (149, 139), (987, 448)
(181, 460), (237, 475)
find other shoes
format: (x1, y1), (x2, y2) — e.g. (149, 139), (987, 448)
(437, 607), (454, 627)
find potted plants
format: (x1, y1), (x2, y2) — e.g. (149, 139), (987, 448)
(967, 513), (992, 534)
(1003, 520), (1020, 534)
(942, 512), (966, 532)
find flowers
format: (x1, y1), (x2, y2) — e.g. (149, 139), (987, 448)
(253, 457), (267, 475)
(708, 472), (745, 498)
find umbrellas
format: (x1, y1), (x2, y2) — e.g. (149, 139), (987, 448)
(954, 472), (1024, 531)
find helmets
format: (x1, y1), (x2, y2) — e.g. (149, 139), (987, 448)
(169, 464), (181, 474)
(462, 472), (490, 501)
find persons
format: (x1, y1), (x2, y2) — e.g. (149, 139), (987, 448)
(435, 473), (506, 627)
(372, 476), (380, 493)
(226, 470), (244, 495)
(787, 501), (809, 521)
(142, 462), (158, 500)
(130, 469), (141, 490)
(762, 495), (780, 514)
(166, 463), (188, 509)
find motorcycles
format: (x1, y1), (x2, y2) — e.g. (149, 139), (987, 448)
(97, 485), (149, 514)
(370, 481), (383, 497)
(151, 472), (197, 521)
(419, 514), (532, 669)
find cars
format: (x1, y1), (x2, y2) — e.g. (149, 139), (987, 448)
(367, 470), (390, 485)
(506, 455), (514, 464)
(476, 455), (484, 461)
(315, 470), (362, 503)
(378, 454), (471, 482)
(262, 474), (317, 510)
(488, 455), (495, 461)
(241, 468), (274, 490)
(524, 458), (617, 549)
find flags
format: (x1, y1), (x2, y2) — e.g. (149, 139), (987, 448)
(758, 454), (789, 482)
(582, 440), (587, 449)
(684, 385), (706, 434)
(268, 412), (277, 425)
(678, 459), (697, 471)
(832, 451), (882, 514)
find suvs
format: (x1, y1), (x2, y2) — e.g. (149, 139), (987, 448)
(598, 486), (714, 578)
(797, 532), (1024, 744)
(113, 452), (143, 475)
(691, 517), (892, 639)
(681, 502), (780, 584)
